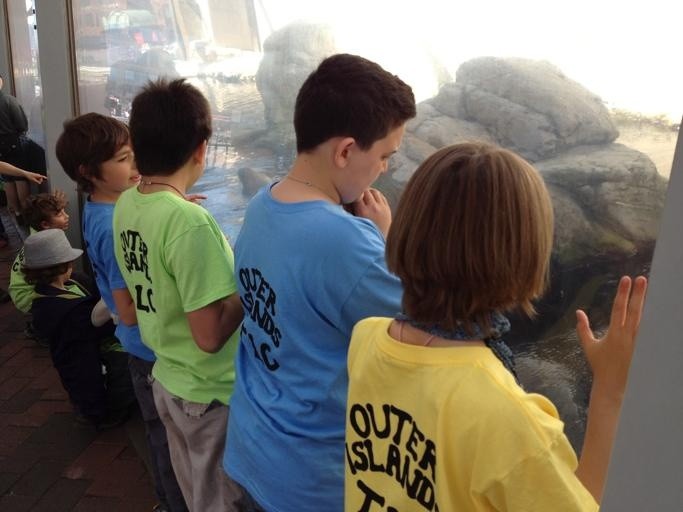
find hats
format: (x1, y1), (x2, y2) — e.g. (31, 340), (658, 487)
(19, 228), (84, 272)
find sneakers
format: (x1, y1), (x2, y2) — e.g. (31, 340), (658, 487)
(78, 410), (129, 430)
(22, 320), (48, 347)
(0, 288), (9, 305)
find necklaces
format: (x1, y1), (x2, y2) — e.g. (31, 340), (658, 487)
(140, 179), (187, 200)
(286, 175), (339, 203)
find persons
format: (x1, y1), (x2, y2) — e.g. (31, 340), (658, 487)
(0, 161), (49, 304)
(7, 189), (86, 349)
(53, 111), (207, 512)
(219, 54), (419, 511)
(17, 229), (133, 430)
(111, 74), (259, 512)
(0, 76), (34, 226)
(342, 139), (647, 511)
